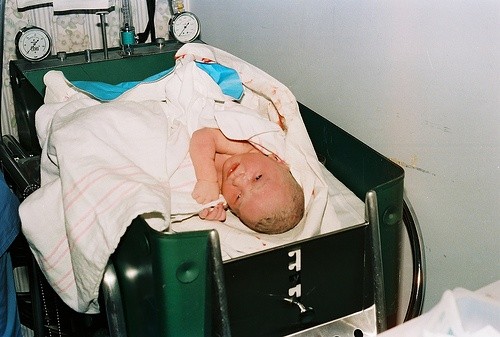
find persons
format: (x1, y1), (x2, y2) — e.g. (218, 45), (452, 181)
(17, 43), (328, 315)
